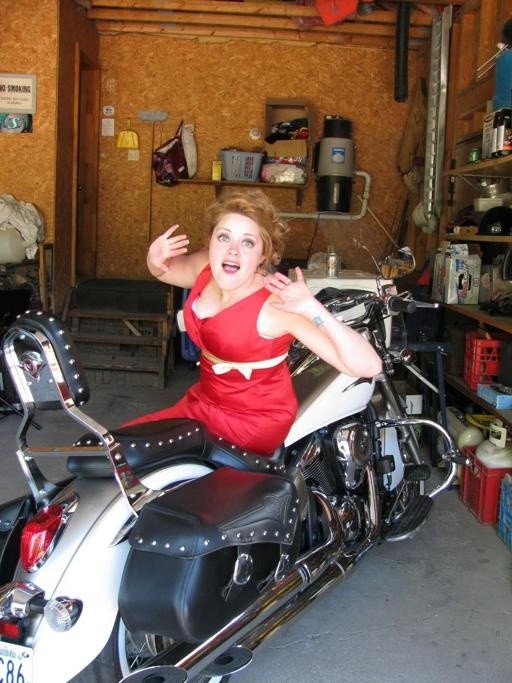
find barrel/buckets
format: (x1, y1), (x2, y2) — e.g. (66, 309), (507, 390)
(317, 137), (357, 178)
(0, 228), (37, 265)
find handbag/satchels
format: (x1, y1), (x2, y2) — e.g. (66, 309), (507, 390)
(153, 120), (189, 188)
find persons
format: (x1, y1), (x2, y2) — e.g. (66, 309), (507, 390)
(119, 187), (385, 481)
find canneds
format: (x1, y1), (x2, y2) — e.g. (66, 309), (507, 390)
(326, 253), (339, 278)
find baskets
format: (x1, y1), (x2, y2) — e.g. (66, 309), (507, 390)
(463, 329), (505, 391)
(219, 150), (265, 182)
(497, 478), (512, 550)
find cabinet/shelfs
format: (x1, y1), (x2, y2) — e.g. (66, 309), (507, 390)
(430, 153), (512, 467)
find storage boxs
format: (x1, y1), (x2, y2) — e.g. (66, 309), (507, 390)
(263, 94), (314, 163)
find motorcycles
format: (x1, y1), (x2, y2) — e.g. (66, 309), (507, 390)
(0, 247), (473, 683)
